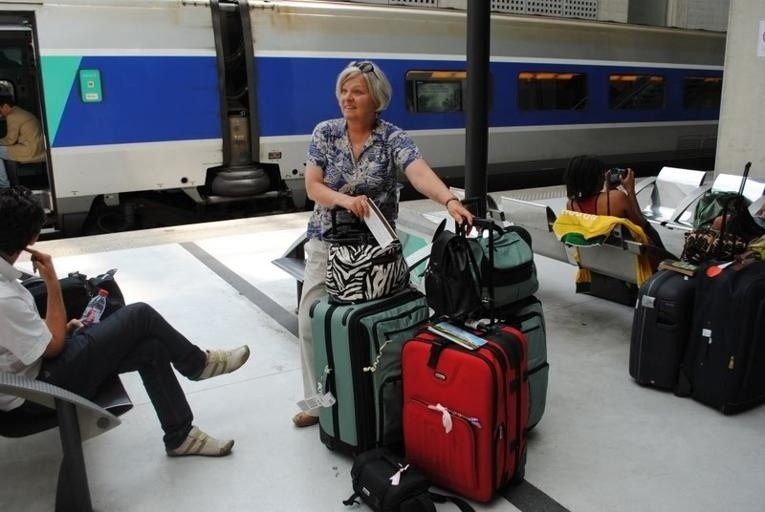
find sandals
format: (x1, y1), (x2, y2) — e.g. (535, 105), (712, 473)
(292, 411), (320, 428)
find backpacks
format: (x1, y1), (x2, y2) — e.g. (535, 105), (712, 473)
(21, 272), (125, 322)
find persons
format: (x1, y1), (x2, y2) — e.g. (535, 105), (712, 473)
(0, 185), (250, 456)
(0, 92), (43, 188)
(291, 58), (476, 427)
(566, 155), (666, 250)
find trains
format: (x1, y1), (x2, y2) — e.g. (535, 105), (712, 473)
(2, 1), (729, 236)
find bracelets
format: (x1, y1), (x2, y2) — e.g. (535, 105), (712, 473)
(445, 196), (460, 209)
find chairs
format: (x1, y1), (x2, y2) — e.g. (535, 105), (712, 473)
(0, 267), (134, 512)
(635, 166), (765, 262)
(499, 195), (680, 308)
(271, 230), (433, 315)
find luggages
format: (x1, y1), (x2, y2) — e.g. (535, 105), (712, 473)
(629, 162), (765, 416)
(309, 204), (551, 512)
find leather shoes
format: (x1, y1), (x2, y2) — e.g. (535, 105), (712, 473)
(165, 425), (235, 458)
(192, 345), (251, 381)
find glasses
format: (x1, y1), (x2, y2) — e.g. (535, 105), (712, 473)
(350, 60), (380, 80)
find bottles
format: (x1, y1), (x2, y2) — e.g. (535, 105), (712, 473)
(71, 288), (109, 335)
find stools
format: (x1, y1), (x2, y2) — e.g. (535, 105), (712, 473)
(11, 152), (46, 186)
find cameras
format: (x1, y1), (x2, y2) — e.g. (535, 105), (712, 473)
(608, 166), (625, 186)
